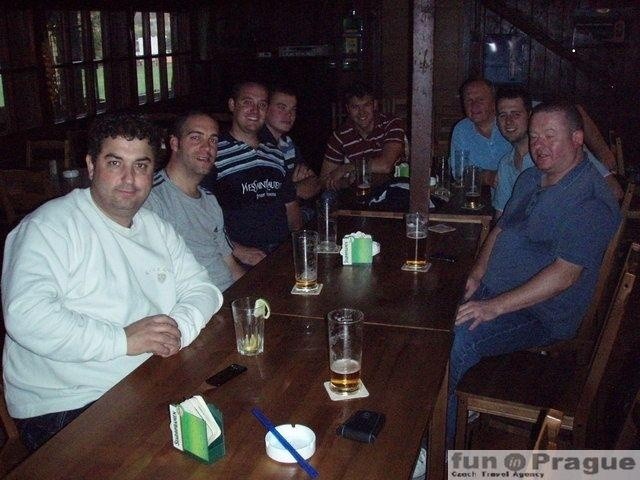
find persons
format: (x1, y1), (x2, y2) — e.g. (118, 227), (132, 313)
(141, 110), (248, 295)
(264, 84), (320, 224)
(446, 77), (619, 193)
(490, 86), (624, 225)
(1, 114), (224, 452)
(209, 76), (304, 268)
(411, 96), (620, 480)
(319, 80), (412, 195)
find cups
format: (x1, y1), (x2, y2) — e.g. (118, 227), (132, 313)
(454, 149), (470, 184)
(462, 164), (481, 207)
(404, 214), (430, 268)
(291, 227), (319, 291)
(315, 197), (340, 252)
(355, 156), (373, 188)
(232, 297), (267, 357)
(326, 307), (364, 395)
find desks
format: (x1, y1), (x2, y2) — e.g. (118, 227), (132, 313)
(0, 177), (495, 480)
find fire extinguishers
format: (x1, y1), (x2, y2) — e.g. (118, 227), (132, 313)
(342, 8), (365, 72)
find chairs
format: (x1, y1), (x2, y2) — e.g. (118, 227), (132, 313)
(0, 112), (233, 228)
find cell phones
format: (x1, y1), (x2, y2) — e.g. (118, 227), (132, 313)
(431, 253), (458, 263)
(206, 363), (247, 387)
